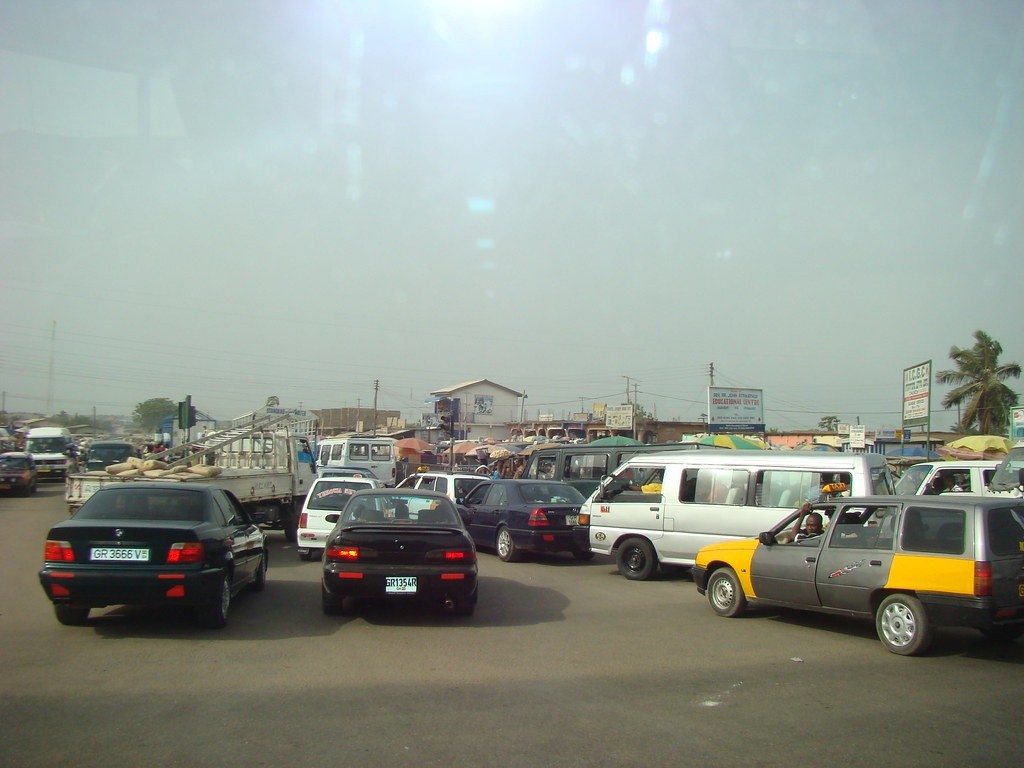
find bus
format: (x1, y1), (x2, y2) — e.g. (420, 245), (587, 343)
(984, 440), (1024, 498)
(12, 418), (46, 435)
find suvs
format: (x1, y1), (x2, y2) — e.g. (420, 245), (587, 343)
(0, 452), (38, 498)
(392, 470), (493, 522)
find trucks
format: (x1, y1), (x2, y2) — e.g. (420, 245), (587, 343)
(0, 428), (18, 450)
(24, 427), (75, 480)
(72, 431), (322, 541)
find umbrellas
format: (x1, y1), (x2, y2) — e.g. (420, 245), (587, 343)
(394, 434), (1013, 464)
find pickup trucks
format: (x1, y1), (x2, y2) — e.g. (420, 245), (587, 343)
(692, 497), (1024, 657)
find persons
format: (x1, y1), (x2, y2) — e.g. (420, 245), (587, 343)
(622, 468), (664, 502)
(703, 475), (728, 503)
(61, 442), (79, 474)
(926, 472), (962, 497)
(143, 443), (171, 463)
(477, 450), (557, 478)
(788, 502), (825, 545)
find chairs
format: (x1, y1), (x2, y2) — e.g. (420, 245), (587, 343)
(361, 510), (385, 521)
(417, 509), (438, 522)
(840, 514), (862, 539)
(939, 523), (963, 552)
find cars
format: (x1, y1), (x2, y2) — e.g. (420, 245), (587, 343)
(38, 483), (270, 629)
(296, 476), (387, 561)
(321, 487), (479, 615)
(87, 440), (145, 472)
(455, 478), (598, 564)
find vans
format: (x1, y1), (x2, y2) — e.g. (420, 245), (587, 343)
(521, 442), (732, 497)
(895, 460), (1023, 496)
(314, 435), (401, 487)
(573, 449), (896, 588)
